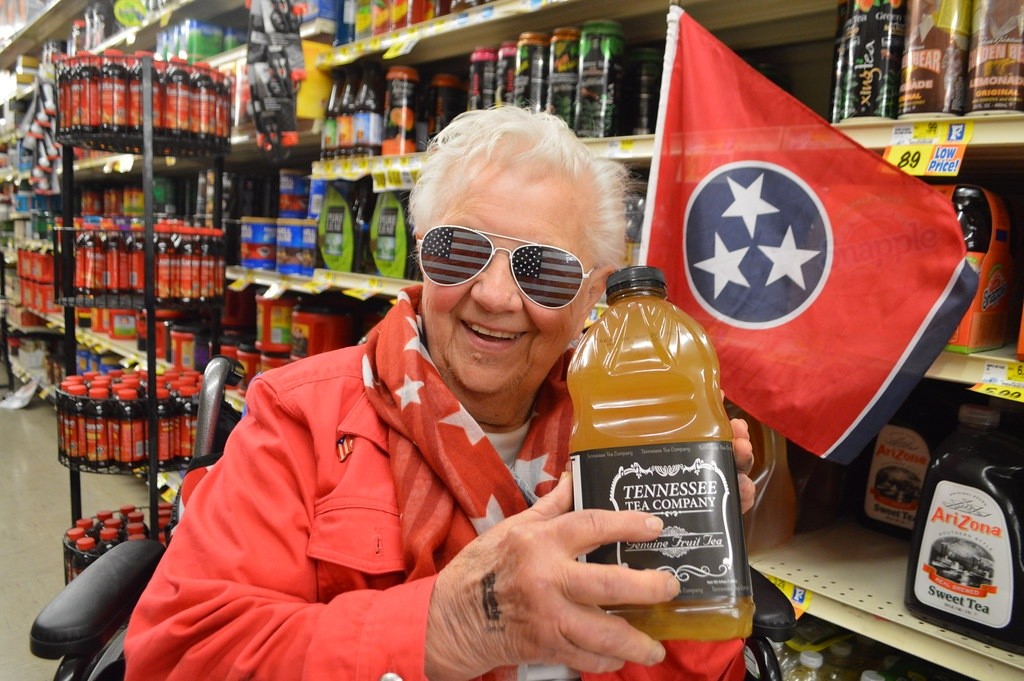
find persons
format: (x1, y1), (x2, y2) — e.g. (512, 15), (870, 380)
(123, 103), (754, 681)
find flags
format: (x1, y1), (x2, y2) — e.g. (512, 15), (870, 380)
(646, 12), (980, 467)
(420, 227), (491, 284)
(512, 246), (581, 308)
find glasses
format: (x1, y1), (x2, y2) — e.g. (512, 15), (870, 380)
(417, 225), (598, 312)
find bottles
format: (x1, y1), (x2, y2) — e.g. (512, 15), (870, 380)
(0, 0), (465, 591)
(743, 608), (970, 681)
(943, 185), (997, 349)
(860, 379), (958, 540)
(620, 170), (649, 268)
(902, 404), (1023, 659)
(560, 265), (757, 637)
